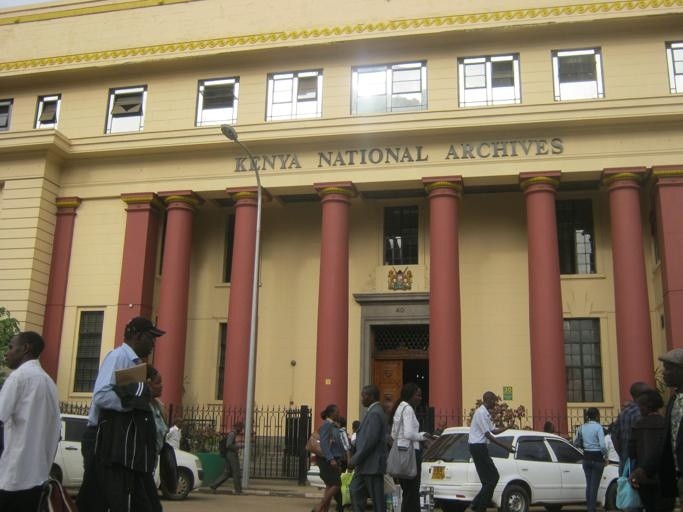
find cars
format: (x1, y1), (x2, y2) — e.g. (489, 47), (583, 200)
(49, 413), (203, 499)
(420, 426), (625, 509)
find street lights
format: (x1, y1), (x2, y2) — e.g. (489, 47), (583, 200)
(218, 123), (262, 490)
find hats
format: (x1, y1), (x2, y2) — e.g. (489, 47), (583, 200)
(124, 316), (166, 339)
(657, 347), (683, 365)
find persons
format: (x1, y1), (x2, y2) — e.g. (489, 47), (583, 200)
(573, 348), (683, 512)
(76, 317), (165, 511)
(146, 363), (183, 512)
(311, 382), (437, 512)
(211, 421), (247, 495)
(468, 391), (516, 512)
(0, 332), (62, 512)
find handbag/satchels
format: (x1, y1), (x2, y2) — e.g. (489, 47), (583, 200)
(340, 472), (354, 506)
(386, 440), (418, 480)
(157, 443), (181, 499)
(304, 424), (334, 456)
(616, 473), (645, 511)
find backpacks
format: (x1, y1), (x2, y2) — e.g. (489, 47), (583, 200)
(219, 438), (226, 458)
